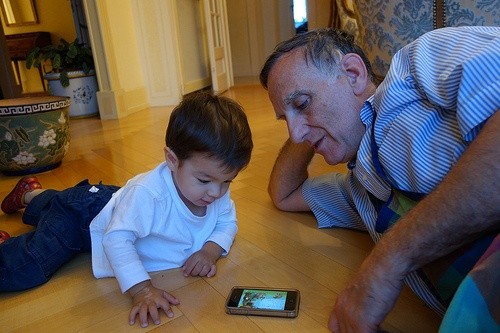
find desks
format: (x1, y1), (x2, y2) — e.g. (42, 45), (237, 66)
(5, 31), (57, 94)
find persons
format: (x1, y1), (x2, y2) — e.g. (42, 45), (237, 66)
(0, 92), (253, 328)
(259, 26), (500, 333)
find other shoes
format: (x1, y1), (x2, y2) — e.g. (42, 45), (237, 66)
(0, 230), (11, 244)
(1, 174), (43, 215)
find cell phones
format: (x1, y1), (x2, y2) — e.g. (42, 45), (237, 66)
(225, 286), (300, 318)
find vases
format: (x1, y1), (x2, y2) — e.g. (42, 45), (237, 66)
(0, 96), (72, 177)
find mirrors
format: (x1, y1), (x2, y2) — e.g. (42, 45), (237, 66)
(0, 0), (39, 27)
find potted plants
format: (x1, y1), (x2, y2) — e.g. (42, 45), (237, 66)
(26, 38), (99, 120)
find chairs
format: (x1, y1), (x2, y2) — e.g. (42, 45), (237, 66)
(334, 0), (500, 87)
